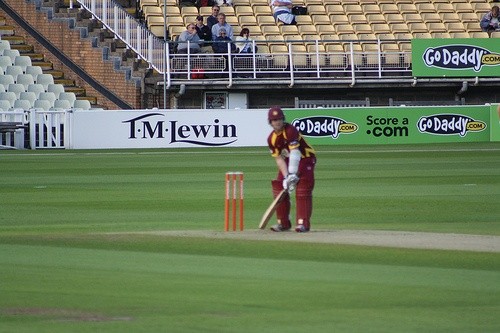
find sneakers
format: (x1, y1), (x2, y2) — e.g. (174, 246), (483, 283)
(295, 225), (308, 232)
(270, 225), (287, 231)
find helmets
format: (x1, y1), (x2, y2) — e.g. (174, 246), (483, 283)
(268, 107), (284, 120)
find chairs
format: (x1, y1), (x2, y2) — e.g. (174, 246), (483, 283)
(140, 0), (500, 67)
(0, 37), (104, 111)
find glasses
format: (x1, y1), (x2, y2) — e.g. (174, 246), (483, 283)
(198, 19), (203, 21)
(214, 9), (219, 12)
(189, 27), (196, 29)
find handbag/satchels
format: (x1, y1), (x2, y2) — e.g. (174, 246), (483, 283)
(292, 6), (309, 15)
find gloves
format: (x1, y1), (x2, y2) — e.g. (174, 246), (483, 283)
(283, 174), (299, 193)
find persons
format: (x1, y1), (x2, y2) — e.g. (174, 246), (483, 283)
(175, 0), (258, 78)
(267, 107), (317, 233)
(480, 5), (500, 38)
(269, 0), (293, 19)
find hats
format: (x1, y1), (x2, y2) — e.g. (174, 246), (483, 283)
(196, 16), (203, 19)
